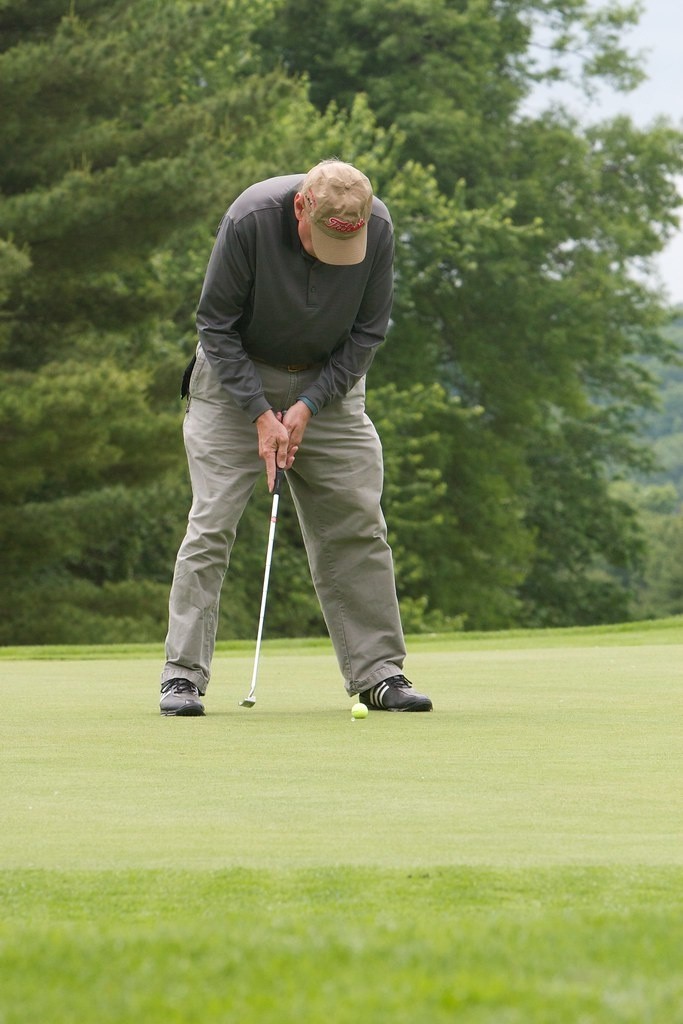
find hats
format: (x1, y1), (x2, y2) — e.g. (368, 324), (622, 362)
(302, 160), (373, 266)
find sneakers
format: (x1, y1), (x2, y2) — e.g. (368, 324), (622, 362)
(359, 675), (432, 712)
(160, 678), (205, 716)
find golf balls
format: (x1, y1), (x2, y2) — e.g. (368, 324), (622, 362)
(350, 702), (368, 720)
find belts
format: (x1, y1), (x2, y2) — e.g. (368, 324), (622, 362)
(249, 356), (323, 372)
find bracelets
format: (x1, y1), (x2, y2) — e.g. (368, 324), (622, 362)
(297, 396), (317, 415)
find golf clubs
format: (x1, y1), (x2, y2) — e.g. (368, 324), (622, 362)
(237, 409), (289, 709)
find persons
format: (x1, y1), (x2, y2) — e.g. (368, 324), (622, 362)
(160, 160), (432, 716)
